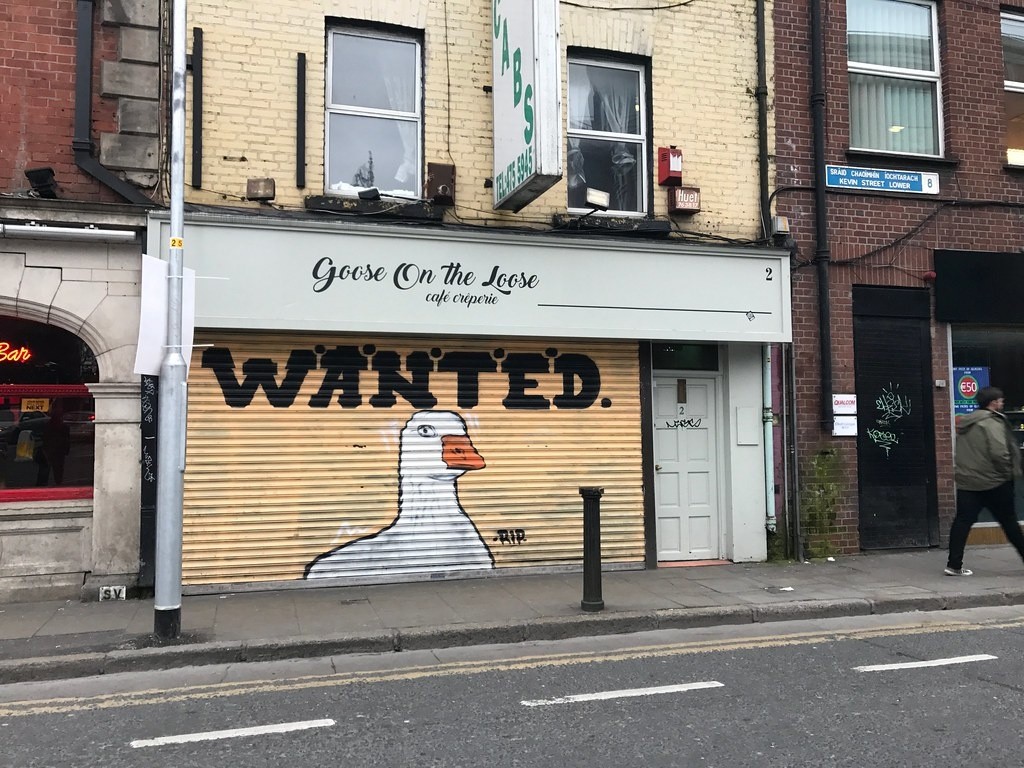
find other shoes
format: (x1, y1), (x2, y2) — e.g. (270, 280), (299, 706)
(944, 567), (973, 576)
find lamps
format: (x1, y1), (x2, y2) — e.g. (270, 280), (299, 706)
(246, 178), (276, 201)
(24, 165), (57, 198)
(580, 187), (611, 220)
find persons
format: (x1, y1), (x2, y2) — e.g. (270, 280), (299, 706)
(943, 386), (1024, 577)
